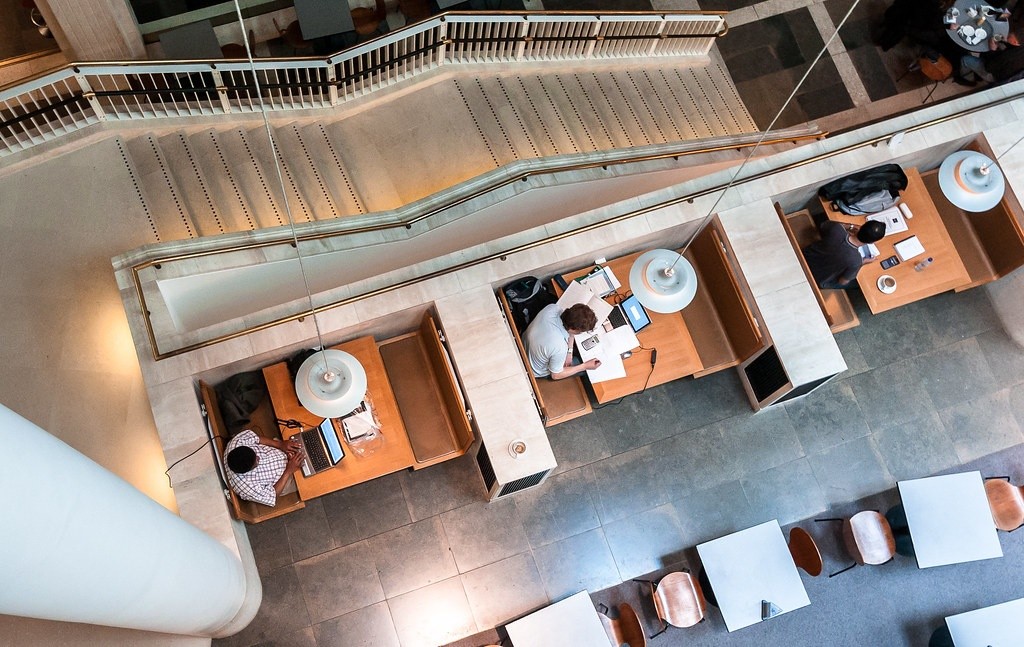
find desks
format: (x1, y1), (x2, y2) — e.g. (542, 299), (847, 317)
(505, 589), (611, 647)
(820, 166), (972, 315)
(943, 0), (1009, 53)
(260, 332), (416, 501)
(552, 249), (704, 405)
(696, 519), (811, 634)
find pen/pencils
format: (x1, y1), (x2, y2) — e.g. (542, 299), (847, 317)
(595, 361), (600, 362)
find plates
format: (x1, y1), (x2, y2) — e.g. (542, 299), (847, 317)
(974, 28), (987, 39)
(984, 6), (996, 18)
(877, 275), (897, 294)
(962, 25), (974, 36)
(946, 14), (956, 23)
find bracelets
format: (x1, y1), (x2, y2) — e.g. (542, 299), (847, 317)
(850, 224), (853, 230)
(567, 348), (572, 352)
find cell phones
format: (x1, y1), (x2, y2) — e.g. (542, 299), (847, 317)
(880, 256), (900, 270)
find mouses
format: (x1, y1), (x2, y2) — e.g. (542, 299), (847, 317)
(621, 351), (632, 359)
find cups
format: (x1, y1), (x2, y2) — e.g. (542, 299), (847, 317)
(993, 34), (1003, 43)
(980, 5), (990, 13)
(952, 8), (960, 17)
(882, 277), (896, 291)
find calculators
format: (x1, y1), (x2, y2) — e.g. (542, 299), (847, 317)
(880, 255), (900, 270)
(581, 334), (601, 351)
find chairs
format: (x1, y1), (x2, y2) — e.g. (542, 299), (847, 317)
(816, 510), (897, 577)
(984, 474), (1024, 534)
(634, 568), (708, 639)
(897, 53), (951, 104)
(895, 469), (1002, 569)
(785, 526), (822, 578)
(597, 602), (646, 647)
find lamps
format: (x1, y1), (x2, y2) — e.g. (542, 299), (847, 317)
(938, 136), (1024, 212)
(231, 0), (368, 419)
(628, 0), (860, 315)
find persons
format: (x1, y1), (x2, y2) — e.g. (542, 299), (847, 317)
(522, 303), (602, 380)
(224, 430), (307, 507)
(804, 220), (886, 289)
(955, 35), (1024, 87)
(926, 0), (958, 31)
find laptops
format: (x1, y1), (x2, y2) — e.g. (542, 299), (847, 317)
(289, 418), (346, 478)
(602, 293), (653, 335)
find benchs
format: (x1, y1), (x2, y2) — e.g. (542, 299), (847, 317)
(196, 139), (1024, 525)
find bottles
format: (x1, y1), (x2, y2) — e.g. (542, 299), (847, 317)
(977, 17), (986, 26)
(915, 257), (933, 271)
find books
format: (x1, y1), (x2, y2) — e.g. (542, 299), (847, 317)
(893, 235), (925, 262)
